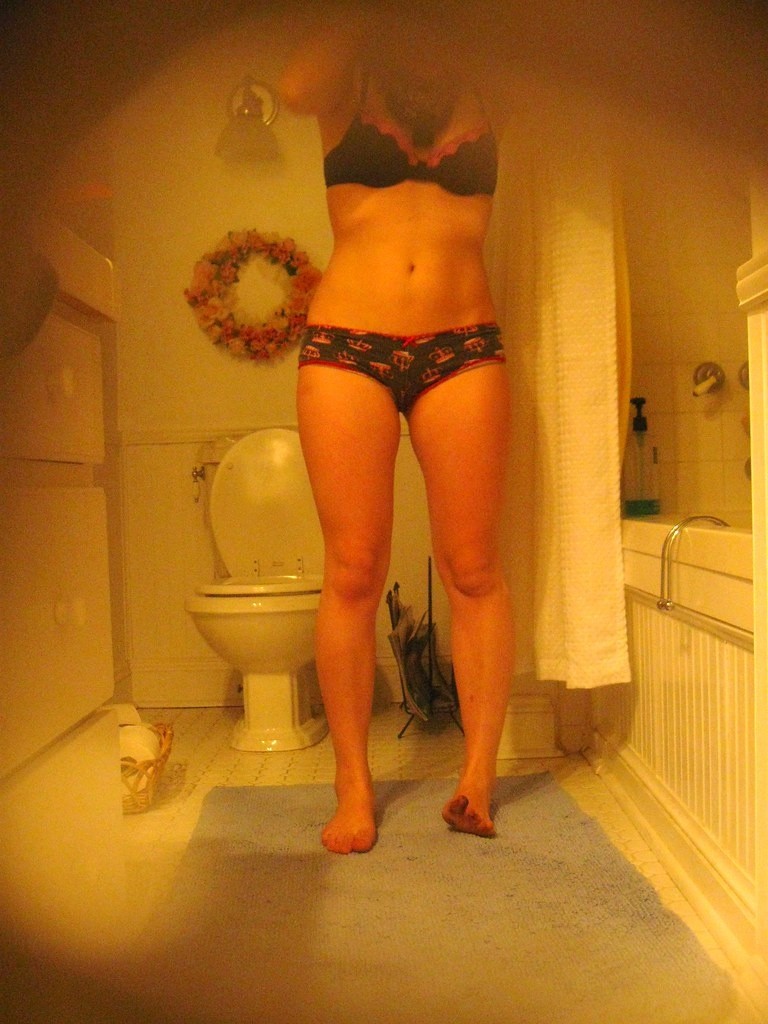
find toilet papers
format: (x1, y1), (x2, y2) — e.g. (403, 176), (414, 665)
(103, 703), (160, 795)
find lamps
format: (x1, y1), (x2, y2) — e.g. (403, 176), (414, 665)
(211, 75), (282, 161)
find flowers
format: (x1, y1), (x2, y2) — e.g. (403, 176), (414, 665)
(181, 228), (323, 362)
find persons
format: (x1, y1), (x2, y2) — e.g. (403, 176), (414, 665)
(284, 27), (514, 854)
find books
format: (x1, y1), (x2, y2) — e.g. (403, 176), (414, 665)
(387, 604), (436, 722)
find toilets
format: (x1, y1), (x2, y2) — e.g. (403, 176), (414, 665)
(181, 424), (325, 753)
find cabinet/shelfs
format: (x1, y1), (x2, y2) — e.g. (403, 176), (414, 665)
(0, 211), (121, 784)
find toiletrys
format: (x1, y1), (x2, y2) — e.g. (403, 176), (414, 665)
(620, 396), (661, 516)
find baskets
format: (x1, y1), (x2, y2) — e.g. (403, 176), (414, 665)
(120, 722), (174, 815)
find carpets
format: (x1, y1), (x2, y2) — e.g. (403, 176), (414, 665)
(126, 768), (738, 1024)
